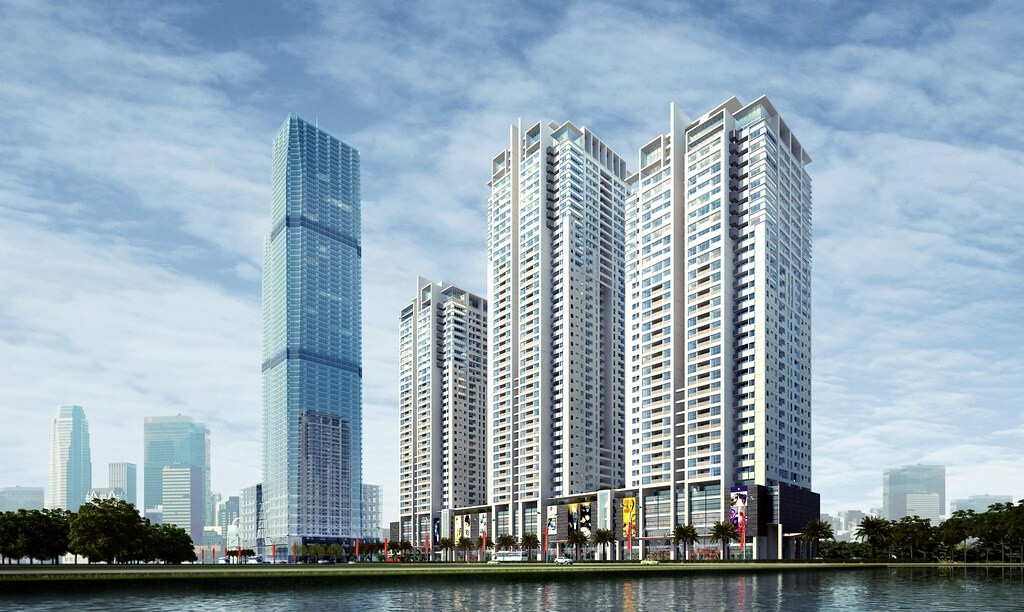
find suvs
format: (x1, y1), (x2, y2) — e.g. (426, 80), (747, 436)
(554, 557), (573, 565)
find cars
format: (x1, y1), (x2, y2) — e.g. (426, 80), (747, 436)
(641, 558), (659, 565)
(318, 559), (329, 564)
(348, 561), (356, 565)
(487, 560), (500, 565)
(297, 560), (305, 564)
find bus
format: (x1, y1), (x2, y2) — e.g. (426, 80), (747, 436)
(248, 556), (262, 565)
(492, 551), (528, 562)
(219, 557), (229, 565)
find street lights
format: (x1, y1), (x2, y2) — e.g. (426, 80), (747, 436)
(621, 504), (642, 560)
(473, 517), (495, 561)
(535, 511), (559, 564)
